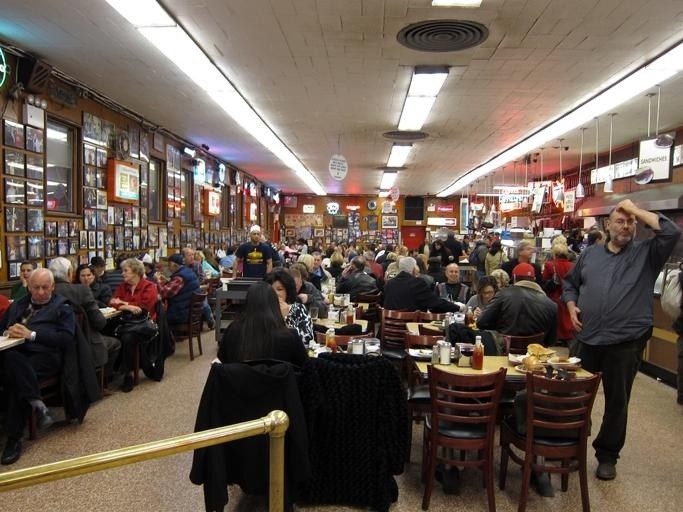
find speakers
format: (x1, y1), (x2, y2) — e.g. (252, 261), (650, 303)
(404, 197), (424, 220)
(20, 58), (52, 94)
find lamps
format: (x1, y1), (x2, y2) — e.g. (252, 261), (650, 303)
(449, 90), (679, 231)
(377, 64), (448, 198)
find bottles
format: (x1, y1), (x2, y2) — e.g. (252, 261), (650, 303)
(327, 294), (356, 326)
(325, 327), (335, 355)
(430, 336), (484, 370)
(344, 337), (380, 356)
(440, 306), (473, 330)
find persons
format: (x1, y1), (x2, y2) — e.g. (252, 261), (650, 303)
(232, 224), (272, 279)
(656, 253), (682, 414)
(560, 198), (682, 480)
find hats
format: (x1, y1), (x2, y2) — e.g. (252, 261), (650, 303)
(162, 253), (185, 266)
(248, 224), (260, 234)
(512, 262), (535, 280)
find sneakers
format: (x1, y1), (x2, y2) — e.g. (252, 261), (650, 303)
(120, 373), (134, 394)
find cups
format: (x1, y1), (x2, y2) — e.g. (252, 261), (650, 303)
(309, 303), (318, 321)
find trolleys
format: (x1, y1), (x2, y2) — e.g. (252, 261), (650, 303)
(210, 275), (264, 344)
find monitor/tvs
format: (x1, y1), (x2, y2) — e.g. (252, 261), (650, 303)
(333, 215), (348, 228)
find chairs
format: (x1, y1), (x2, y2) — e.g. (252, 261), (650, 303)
(307, 254), (602, 510)
(2, 257), (244, 440)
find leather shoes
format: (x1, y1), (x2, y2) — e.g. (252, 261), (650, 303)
(30, 405), (58, 440)
(0, 435), (25, 465)
(595, 459), (617, 480)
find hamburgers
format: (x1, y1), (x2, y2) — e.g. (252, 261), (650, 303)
(521, 355), (544, 372)
(527, 344), (555, 362)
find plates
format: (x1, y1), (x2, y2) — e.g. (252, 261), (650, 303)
(507, 352), (581, 375)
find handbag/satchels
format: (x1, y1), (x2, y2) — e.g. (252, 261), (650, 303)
(112, 309), (158, 346)
(538, 255), (564, 293)
